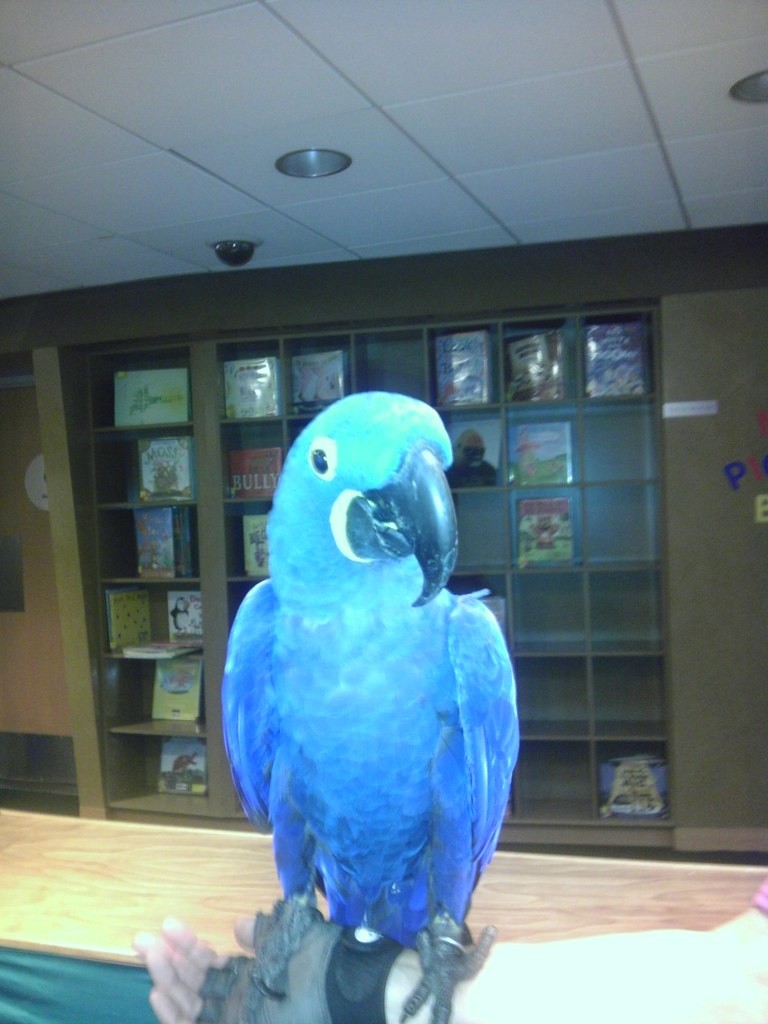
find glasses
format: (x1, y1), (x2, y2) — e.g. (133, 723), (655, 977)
(461, 444), (486, 457)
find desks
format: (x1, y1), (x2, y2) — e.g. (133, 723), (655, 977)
(0, 808), (768, 1024)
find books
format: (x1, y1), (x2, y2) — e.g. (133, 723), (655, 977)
(585, 321), (648, 396)
(134, 506), (192, 578)
(158, 735), (207, 796)
(517, 496), (574, 562)
(435, 331), (492, 405)
(444, 420), (502, 489)
(113, 369), (190, 424)
(230, 447), (281, 499)
(152, 654), (202, 721)
(168, 590), (202, 642)
(509, 421), (574, 486)
(598, 755), (668, 820)
(224, 357), (279, 418)
(243, 514), (268, 576)
(137, 437), (193, 502)
(125, 643), (198, 659)
(290, 349), (349, 414)
(508, 329), (568, 402)
(105, 586), (151, 655)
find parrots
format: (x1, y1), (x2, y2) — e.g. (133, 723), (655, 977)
(220, 390), (521, 1023)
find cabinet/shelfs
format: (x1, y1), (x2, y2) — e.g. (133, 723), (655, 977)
(85, 301), (675, 847)
